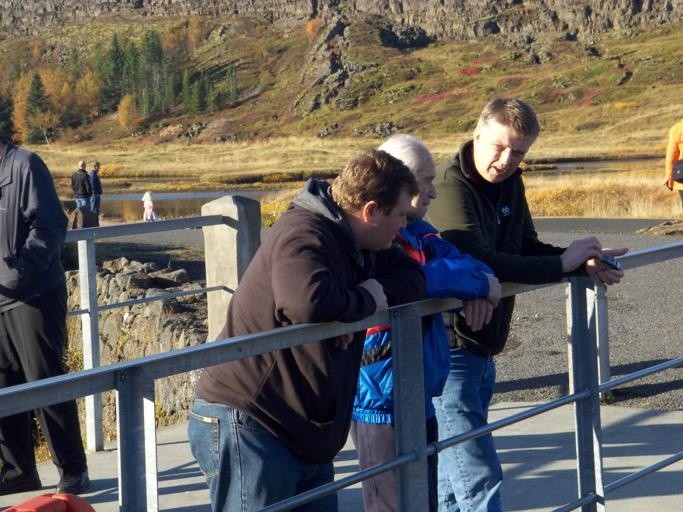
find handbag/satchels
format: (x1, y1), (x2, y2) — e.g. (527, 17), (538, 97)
(671, 159), (683, 182)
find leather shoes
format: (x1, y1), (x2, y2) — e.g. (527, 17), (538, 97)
(0, 464), (40, 496)
(55, 464), (89, 494)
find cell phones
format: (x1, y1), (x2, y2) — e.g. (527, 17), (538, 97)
(592, 255), (621, 271)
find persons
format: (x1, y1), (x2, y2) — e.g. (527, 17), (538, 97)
(427, 96), (628, 511)
(140, 191), (158, 222)
(349, 132), (502, 511)
(663, 120), (682, 223)
(0, 87), (90, 495)
(87, 161), (103, 226)
(184, 148), (415, 511)
(70, 161), (91, 230)
